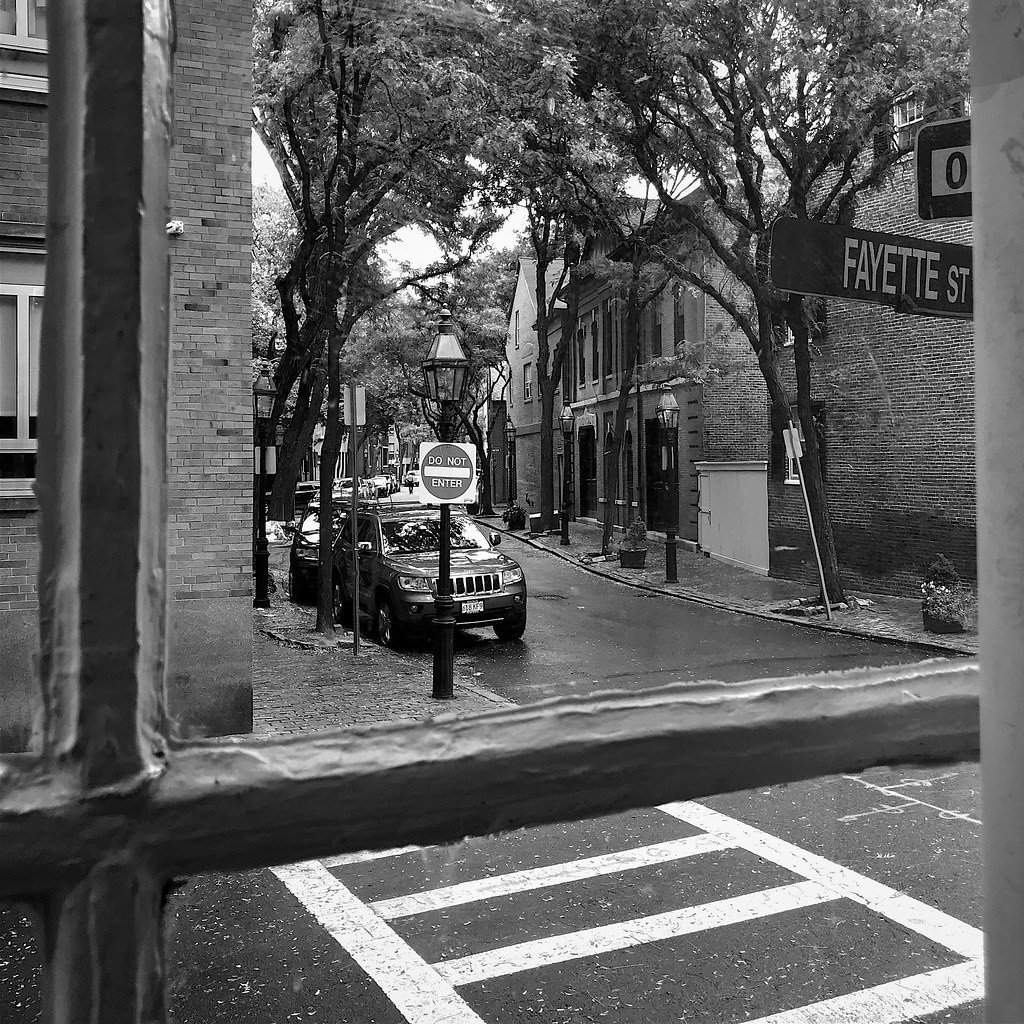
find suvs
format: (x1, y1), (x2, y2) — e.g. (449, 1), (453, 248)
(330, 499), (527, 649)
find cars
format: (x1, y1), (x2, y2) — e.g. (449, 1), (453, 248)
(405, 470), (420, 487)
(267, 472), (401, 601)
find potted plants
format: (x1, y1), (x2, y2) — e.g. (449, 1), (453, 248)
(921, 551), (977, 633)
(501, 499), (526, 530)
(619, 515), (648, 568)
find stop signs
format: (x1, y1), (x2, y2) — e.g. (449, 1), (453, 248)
(418, 442), (477, 504)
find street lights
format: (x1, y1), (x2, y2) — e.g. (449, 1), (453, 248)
(558, 395), (575, 545)
(419, 304), (470, 700)
(253, 362), (278, 609)
(502, 413), (517, 508)
(655, 383), (680, 583)
